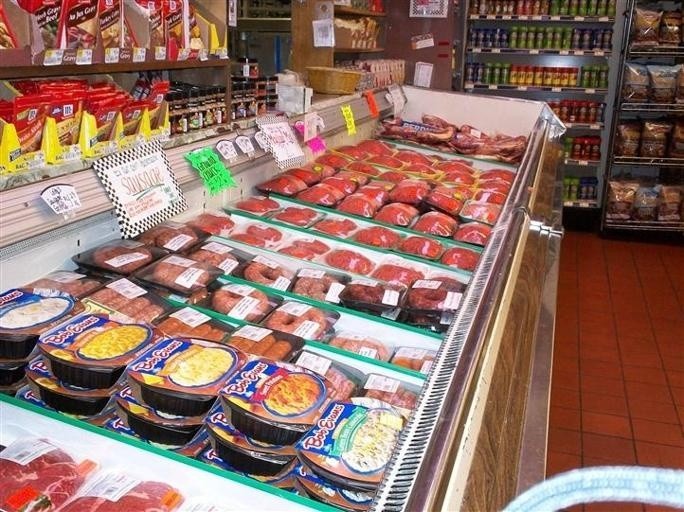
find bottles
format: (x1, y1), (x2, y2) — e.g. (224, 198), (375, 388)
(548, 99), (606, 201)
(463, 0), (617, 89)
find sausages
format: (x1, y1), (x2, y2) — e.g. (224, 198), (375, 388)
(20, 220), (451, 410)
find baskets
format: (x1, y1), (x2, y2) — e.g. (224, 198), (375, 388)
(305, 66), (362, 95)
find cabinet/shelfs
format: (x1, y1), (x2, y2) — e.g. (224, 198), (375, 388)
(0, 0), (232, 193)
(463, 0), (629, 209)
(291, 0), (388, 86)
(600, 0), (684, 237)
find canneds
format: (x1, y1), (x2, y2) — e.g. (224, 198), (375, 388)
(469, 1), (616, 16)
(546, 100), (607, 123)
(562, 175), (599, 201)
(560, 134), (602, 161)
(162, 58), (278, 136)
(467, 26), (614, 50)
(463, 61), (610, 89)
